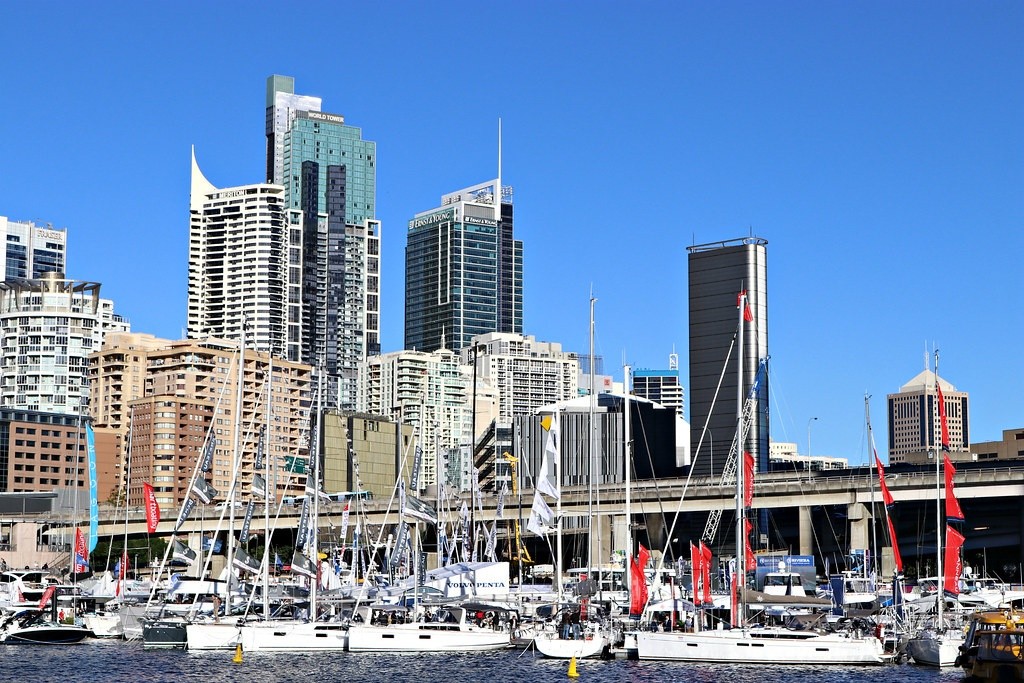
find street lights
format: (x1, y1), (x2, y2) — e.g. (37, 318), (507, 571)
(807, 416), (818, 483)
(703, 426), (714, 486)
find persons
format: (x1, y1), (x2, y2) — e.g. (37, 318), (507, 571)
(58, 608), (85, 627)
(922, 626), (935, 639)
(211, 595), (221, 623)
(559, 607), (580, 641)
(651, 615), (870, 637)
(318, 604), (517, 640)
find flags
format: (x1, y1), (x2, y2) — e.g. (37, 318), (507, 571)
(730, 571), (744, 629)
(943, 524), (965, 599)
(526, 412), (560, 540)
(41, 528), (130, 610)
(692, 544), (701, 605)
(390, 446), (532, 585)
(745, 519), (756, 571)
(938, 383), (950, 453)
(83, 423), (97, 554)
(744, 451), (754, 506)
(579, 544), (650, 620)
(701, 542), (712, 603)
(871, 434), (895, 509)
(886, 510), (904, 574)
(746, 357), (768, 402)
(143, 427), (367, 579)
(737, 290), (754, 321)
(944, 453), (965, 523)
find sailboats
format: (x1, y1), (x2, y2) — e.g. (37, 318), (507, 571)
(0, 283), (1024, 683)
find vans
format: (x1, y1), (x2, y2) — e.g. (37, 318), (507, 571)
(215, 500), (246, 510)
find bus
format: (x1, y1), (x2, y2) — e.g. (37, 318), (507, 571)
(284, 491), (373, 504)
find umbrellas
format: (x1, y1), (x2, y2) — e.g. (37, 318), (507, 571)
(648, 598), (696, 633)
